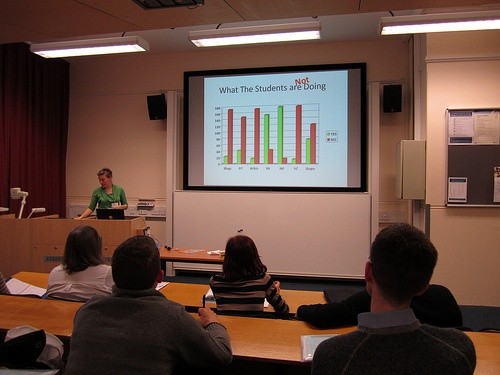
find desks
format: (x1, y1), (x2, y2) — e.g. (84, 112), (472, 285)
(1, 214), (500, 375)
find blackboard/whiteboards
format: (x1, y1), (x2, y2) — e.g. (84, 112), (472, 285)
(444, 105), (500, 208)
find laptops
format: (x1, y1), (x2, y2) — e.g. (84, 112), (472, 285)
(96, 208), (125, 220)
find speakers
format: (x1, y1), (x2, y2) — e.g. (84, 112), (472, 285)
(383, 84), (403, 113)
(147, 94), (167, 120)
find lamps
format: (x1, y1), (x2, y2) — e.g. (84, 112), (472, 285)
(30, 36), (150, 59)
(377, 10), (500, 36)
(11, 188), (28, 219)
(27, 208), (45, 219)
(188, 21), (321, 48)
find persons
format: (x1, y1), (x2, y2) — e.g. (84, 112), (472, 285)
(209, 235), (289, 318)
(74, 168), (128, 220)
(47, 225), (116, 302)
(293, 284), (464, 329)
(63, 235), (233, 375)
(312, 222), (476, 375)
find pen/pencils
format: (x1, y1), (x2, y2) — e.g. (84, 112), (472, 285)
(203, 294), (206, 308)
